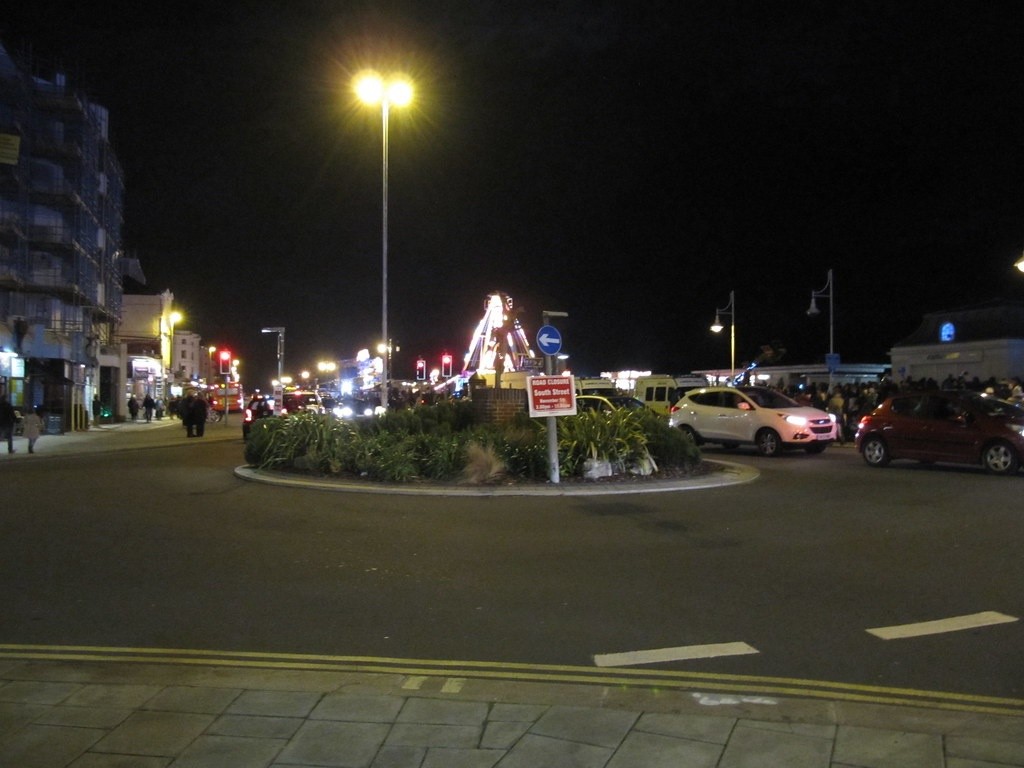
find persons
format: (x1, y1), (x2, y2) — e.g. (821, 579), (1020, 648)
(140, 392), (156, 423)
(0, 394), (22, 453)
(93, 394), (105, 427)
(180, 390), (210, 438)
(127, 394), (142, 421)
(154, 396), (166, 418)
(901, 372), (1024, 405)
(743, 372), (898, 444)
(168, 393), (181, 419)
(22, 403), (44, 454)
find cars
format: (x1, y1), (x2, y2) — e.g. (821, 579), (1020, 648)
(573, 394), (660, 421)
(852, 388), (1024, 477)
(669, 385), (839, 458)
(247, 378), (437, 422)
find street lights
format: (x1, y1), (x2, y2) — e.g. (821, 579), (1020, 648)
(708, 290), (735, 377)
(350, 67), (417, 412)
(807, 267), (839, 398)
(542, 310), (571, 484)
(261, 326), (285, 416)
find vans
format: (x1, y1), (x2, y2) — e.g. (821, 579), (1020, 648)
(634, 373), (710, 416)
(574, 375), (619, 398)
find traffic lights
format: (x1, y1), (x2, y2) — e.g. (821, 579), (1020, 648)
(219, 350), (231, 375)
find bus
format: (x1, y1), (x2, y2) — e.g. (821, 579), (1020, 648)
(210, 382), (244, 412)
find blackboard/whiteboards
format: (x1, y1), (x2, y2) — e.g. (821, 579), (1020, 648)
(46, 416), (63, 434)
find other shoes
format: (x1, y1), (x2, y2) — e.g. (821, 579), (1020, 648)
(9, 450), (15, 454)
(29, 448), (33, 453)
